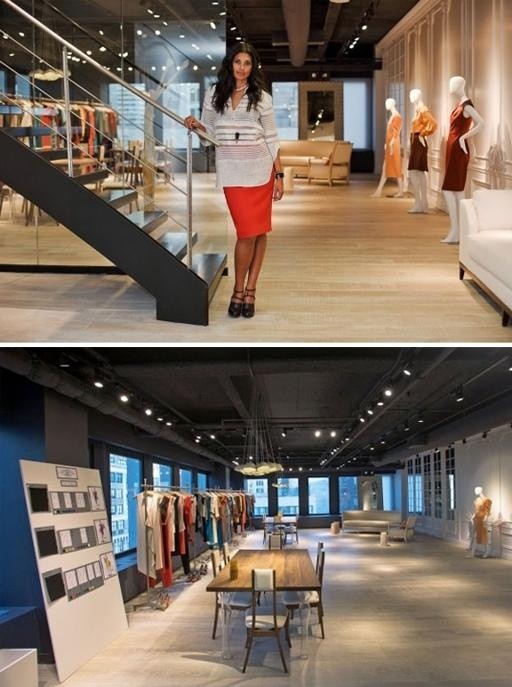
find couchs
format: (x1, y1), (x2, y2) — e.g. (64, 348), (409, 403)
(278, 140), (350, 177)
(453, 187), (512, 328)
(342, 511), (402, 534)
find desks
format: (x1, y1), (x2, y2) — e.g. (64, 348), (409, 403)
(206, 549), (321, 660)
(50, 157), (97, 176)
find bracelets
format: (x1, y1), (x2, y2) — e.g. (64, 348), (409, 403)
(275, 172), (285, 179)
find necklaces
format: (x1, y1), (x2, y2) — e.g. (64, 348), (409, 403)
(232, 84), (250, 91)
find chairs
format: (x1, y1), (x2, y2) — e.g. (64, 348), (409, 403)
(261, 512), (299, 550)
(282, 548), (324, 640)
(285, 540), (326, 619)
(388, 516), (417, 543)
(241, 569), (291, 674)
(211, 549), (260, 640)
(223, 541), (265, 606)
(83, 138), (174, 214)
(307, 141), (354, 186)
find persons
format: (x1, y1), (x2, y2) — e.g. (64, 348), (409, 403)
(404, 89), (438, 214)
(465, 486), (493, 558)
(370, 97), (404, 197)
(181, 40), (285, 317)
(437, 75), (487, 245)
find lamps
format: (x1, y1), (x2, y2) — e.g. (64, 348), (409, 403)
(233, 399), (281, 477)
(29, 0), (71, 82)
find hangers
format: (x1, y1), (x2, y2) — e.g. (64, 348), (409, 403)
(132, 486), (153, 500)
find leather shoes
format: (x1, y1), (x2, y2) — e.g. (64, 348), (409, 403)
(228, 287), (244, 317)
(241, 286), (256, 318)
